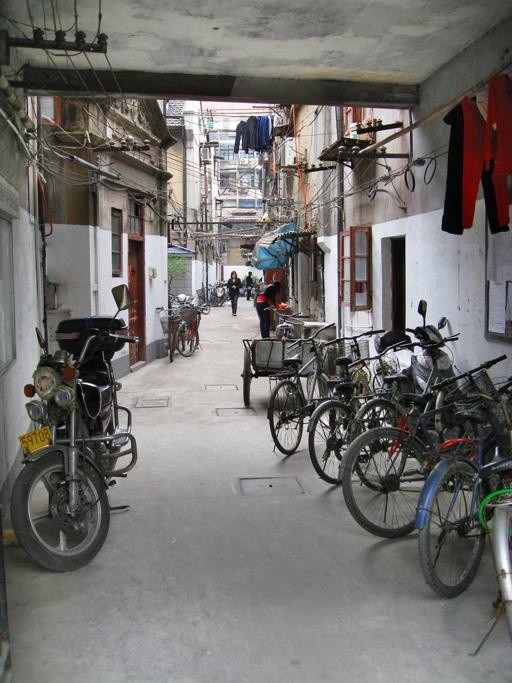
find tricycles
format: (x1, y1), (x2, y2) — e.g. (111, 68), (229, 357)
(241, 307), (329, 405)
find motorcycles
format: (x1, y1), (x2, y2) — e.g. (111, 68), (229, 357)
(12, 286), (136, 570)
(373, 300), (458, 392)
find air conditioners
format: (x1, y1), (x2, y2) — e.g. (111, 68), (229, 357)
(279, 141), (294, 168)
(239, 157), (248, 165)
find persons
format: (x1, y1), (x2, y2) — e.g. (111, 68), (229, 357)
(226, 269), (243, 316)
(254, 280), (282, 339)
(245, 270), (254, 300)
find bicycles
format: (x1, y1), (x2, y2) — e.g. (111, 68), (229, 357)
(252, 282), (263, 306)
(156, 281), (229, 362)
